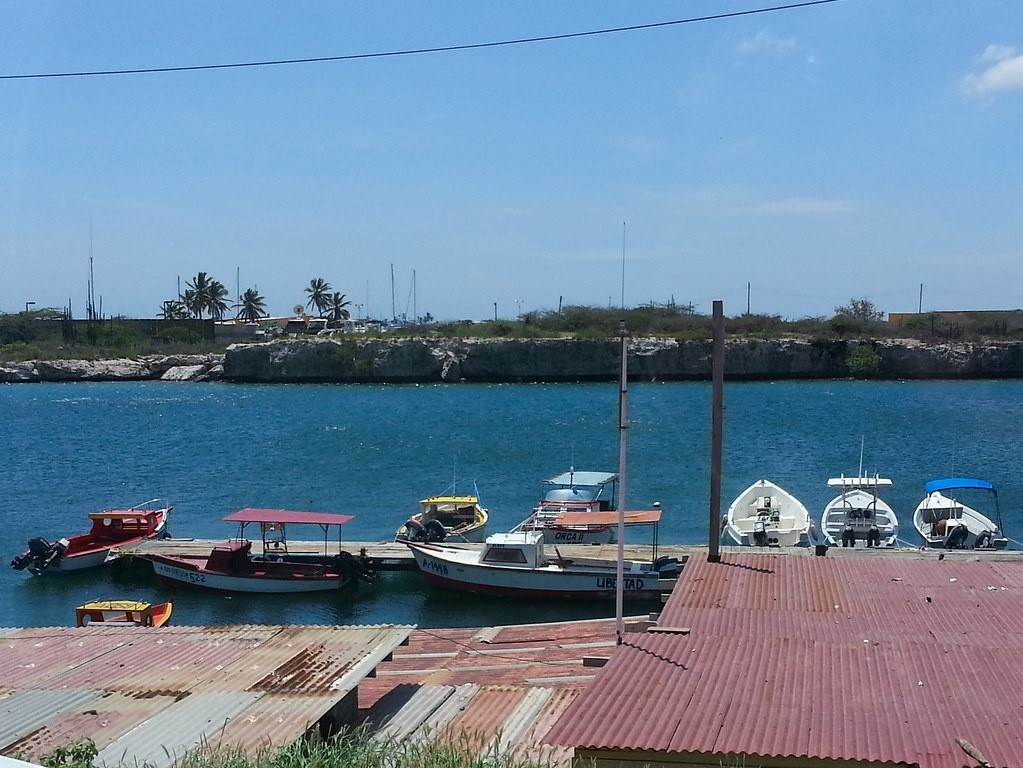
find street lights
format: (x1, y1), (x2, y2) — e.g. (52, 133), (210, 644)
(25, 301), (35, 312)
(514, 299), (525, 318)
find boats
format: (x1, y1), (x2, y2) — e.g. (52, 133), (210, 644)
(522, 444), (622, 546)
(404, 509), (662, 601)
(821, 434), (900, 548)
(145, 508), (380, 594)
(721, 475), (820, 549)
(10, 498), (173, 577)
(911, 434), (1010, 551)
(395, 454), (489, 544)
(74, 597), (174, 628)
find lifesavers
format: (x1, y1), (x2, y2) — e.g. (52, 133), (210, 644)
(405, 519), (447, 540)
